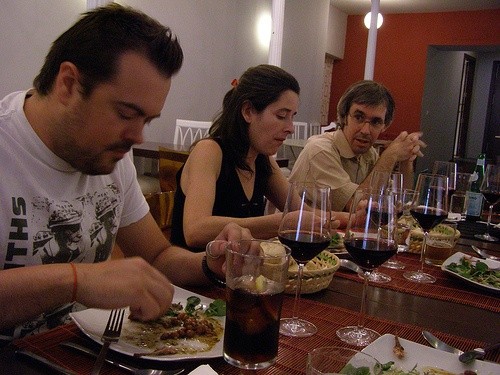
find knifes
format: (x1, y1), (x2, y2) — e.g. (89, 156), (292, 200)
(459, 342), (500, 364)
(472, 245), (500, 259)
(422, 330), (500, 364)
(59, 340), (185, 375)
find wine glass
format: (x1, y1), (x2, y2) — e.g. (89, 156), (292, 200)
(277, 181), (332, 337)
(357, 170), (404, 284)
(336, 190), (398, 346)
(403, 173), (448, 283)
(474, 164), (500, 241)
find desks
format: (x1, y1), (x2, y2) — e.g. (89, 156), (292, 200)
(133, 141), (289, 168)
(284, 139), (307, 161)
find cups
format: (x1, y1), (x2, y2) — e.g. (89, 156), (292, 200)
(223, 240), (291, 370)
(305, 346), (383, 375)
(429, 161), (456, 195)
(450, 173), (474, 221)
(382, 187), (420, 270)
(424, 221), (457, 267)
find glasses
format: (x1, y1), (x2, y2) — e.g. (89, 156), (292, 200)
(348, 112), (387, 130)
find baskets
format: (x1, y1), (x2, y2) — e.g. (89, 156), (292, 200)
(260, 240), (340, 295)
(390, 219), (460, 254)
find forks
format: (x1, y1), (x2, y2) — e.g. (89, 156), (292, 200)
(91, 308), (125, 375)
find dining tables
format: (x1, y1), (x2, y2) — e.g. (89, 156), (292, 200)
(0, 206), (500, 375)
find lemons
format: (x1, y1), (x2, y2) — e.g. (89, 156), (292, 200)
(256, 275), (280, 322)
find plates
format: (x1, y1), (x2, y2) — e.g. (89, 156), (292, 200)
(326, 230), (366, 255)
(69, 284), (225, 361)
(441, 251), (500, 291)
(339, 333), (500, 375)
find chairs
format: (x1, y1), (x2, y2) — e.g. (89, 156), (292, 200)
(143, 146), (191, 245)
(173, 119), (213, 145)
(284, 122), (320, 156)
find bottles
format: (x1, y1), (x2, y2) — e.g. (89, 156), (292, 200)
(462, 153), (485, 221)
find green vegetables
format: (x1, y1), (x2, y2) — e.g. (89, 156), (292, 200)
(444, 258), (500, 290)
(163, 296), (228, 319)
(330, 232), (352, 244)
(339, 362), (428, 375)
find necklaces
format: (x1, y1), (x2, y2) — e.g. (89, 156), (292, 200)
(202, 254), (226, 290)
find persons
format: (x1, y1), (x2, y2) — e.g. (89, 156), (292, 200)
(288, 79), (428, 212)
(0, 3), (265, 350)
(169, 64), (386, 255)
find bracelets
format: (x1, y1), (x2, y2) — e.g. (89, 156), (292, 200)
(70, 262), (78, 303)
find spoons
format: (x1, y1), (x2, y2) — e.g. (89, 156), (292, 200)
(340, 259), (364, 275)
